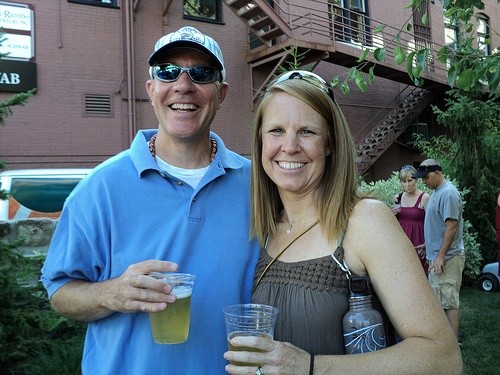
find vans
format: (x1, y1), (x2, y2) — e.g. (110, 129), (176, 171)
(0, 168), (94, 223)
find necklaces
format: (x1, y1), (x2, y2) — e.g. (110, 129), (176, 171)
(283, 209), (314, 234)
(149, 134), (217, 162)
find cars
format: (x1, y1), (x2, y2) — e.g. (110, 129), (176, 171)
(476, 262), (500, 292)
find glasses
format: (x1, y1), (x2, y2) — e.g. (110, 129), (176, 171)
(261, 70), (336, 107)
(152, 62), (221, 84)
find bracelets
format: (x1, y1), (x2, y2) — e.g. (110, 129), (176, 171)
(310, 355), (314, 375)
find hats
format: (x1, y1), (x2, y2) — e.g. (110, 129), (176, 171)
(411, 164), (442, 179)
(147, 26), (226, 84)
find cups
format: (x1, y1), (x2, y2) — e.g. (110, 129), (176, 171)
(222, 304), (279, 367)
(145, 273), (196, 345)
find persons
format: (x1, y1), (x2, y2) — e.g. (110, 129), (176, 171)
(391, 166), (429, 275)
(223, 70), (463, 375)
(411, 158), (465, 339)
(40, 26), (261, 375)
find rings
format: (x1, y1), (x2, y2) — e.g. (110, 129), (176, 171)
(256, 366), (263, 375)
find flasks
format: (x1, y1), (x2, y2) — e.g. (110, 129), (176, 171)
(345, 276), (386, 355)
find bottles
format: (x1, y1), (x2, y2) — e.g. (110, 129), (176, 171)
(429, 268), (441, 288)
